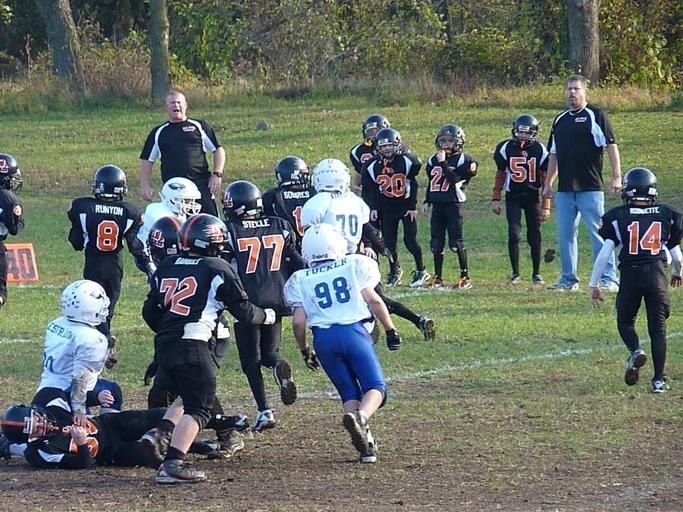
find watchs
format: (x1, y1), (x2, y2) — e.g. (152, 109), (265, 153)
(212, 171), (222, 178)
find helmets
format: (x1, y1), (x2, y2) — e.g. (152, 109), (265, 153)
(179, 213), (231, 257)
(301, 223), (348, 268)
(93, 164), (128, 199)
(159, 176), (202, 216)
(149, 216), (183, 268)
(435, 123), (465, 160)
(60, 280), (111, 327)
(275, 155), (312, 189)
(0, 404), (57, 450)
(621, 167), (658, 204)
(313, 158), (351, 192)
(512, 114), (538, 149)
(362, 114), (390, 148)
(221, 180), (263, 219)
(0, 153), (23, 195)
(374, 128), (404, 160)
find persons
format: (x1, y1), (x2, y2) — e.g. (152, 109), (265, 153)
(140, 88), (226, 220)
(65, 165), (156, 370)
(220, 180), (307, 432)
(136, 176), (202, 279)
(491, 114), (550, 285)
(33, 277), (123, 428)
(2, 386), (248, 470)
(421, 123), (477, 291)
(361, 128), (431, 289)
(589, 167), (683, 395)
(299, 157), (375, 338)
(541, 74), (623, 293)
(349, 114), (397, 263)
(0, 153), (25, 310)
(355, 220), (436, 345)
(262, 155), (317, 314)
(140, 212), (277, 483)
(283, 222), (403, 464)
(148, 218), (246, 460)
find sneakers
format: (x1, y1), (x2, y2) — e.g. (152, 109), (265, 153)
(417, 315), (434, 341)
(251, 408), (275, 431)
(625, 349), (647, 385)
(214, 413), (249, 433)
(423, 274), (445, 288)
(452, 276), (472, 289)
(343, 409), (369, 455)
(196, 440), (221, 455)
(600, 284), (616, 291)
(652, 377), (670, 393)
(360, 438), (378, 463)
(273, 360), (297, 406)
(208, 430), (245, 459)
(408, 265), (432, 288)
(383, 264), (405, 286)
(105, 335), (116, 369)
(532, 274), (546, 285)
(154, 459), (208, 483)
(508, 273), (522, 284)
(556, 282), (579, 292)
(0, 436), (16, 459)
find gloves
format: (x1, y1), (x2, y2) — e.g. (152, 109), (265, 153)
(380, 246), (394, 263)
(386, 329), (403, 350)
(270, 307), (283, 324)
(144, 265), (155, 284)
(300, 345), (321, 372)
